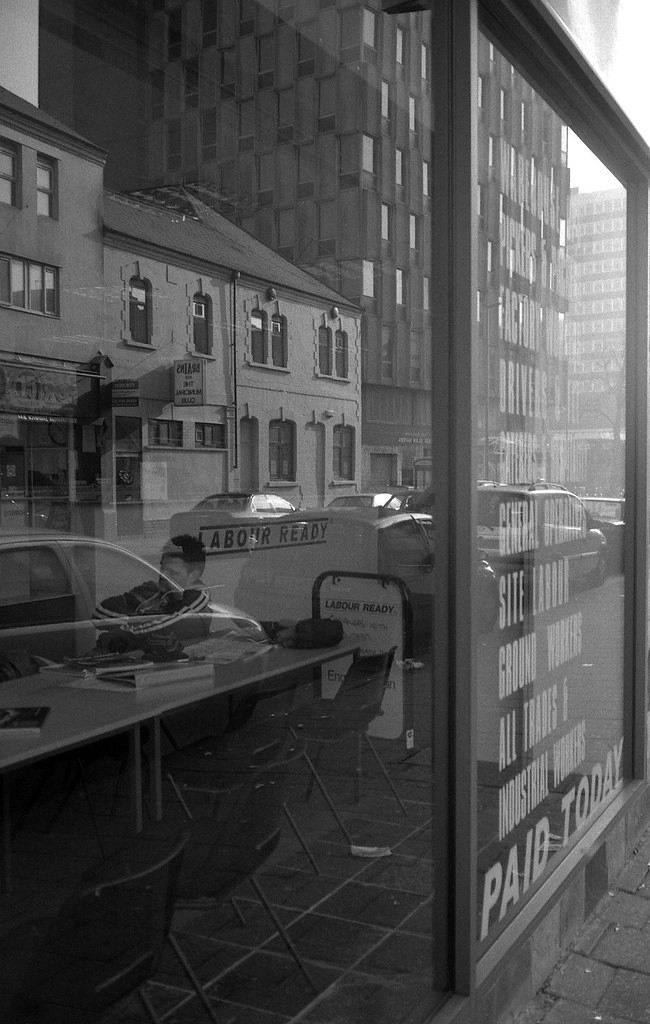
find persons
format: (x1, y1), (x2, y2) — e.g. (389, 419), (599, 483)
(84, 535), (211, 666)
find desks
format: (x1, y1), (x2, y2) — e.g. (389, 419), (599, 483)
(46, 643), (361, 822)
(0, 674), (163, 840)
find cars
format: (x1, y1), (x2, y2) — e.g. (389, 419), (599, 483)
(0, 529), (265, 742)
(168, 491), (296, 555)
(328, 480), (625, 584)
(233, 506), (512, 670)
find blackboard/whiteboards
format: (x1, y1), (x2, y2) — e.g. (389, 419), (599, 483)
(312, 570), (413, 745)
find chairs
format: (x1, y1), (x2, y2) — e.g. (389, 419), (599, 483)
(0, 646), (408, 1024)
